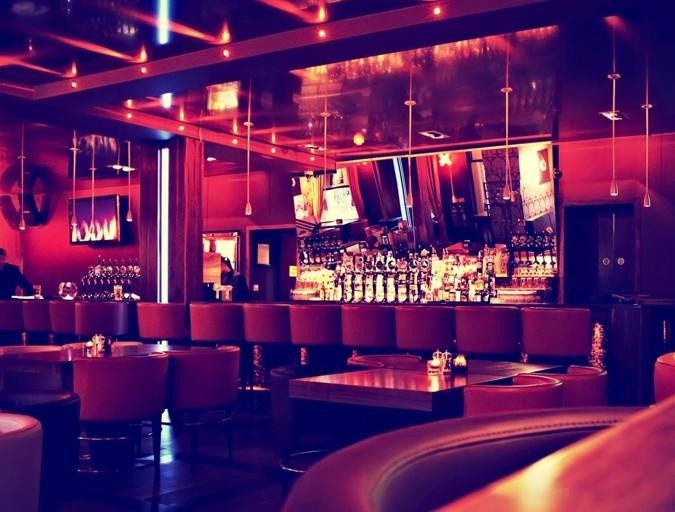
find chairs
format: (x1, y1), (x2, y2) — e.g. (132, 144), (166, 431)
(275, 349), (675, 511)
(0, 333), (241, 511)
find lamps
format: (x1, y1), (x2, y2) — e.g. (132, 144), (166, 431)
(604, 39), (653, 212)
(400, 98), (421, 210)
(240, 116), (261, 217)
(498, 84), (518, 203)
(18, 124), (142, 236)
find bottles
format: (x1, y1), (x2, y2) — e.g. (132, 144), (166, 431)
(77, 254), (141, 301)
(297, 229), (558, 304)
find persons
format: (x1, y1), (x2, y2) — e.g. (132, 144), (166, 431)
(0, 246), (33, 347)
(216, 256), (248, 299)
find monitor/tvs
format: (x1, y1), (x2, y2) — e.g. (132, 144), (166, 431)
(67, 193), (121, 245)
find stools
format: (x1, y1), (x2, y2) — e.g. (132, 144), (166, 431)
(0, 296), (609, 390)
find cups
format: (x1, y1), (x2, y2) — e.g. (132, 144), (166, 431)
(58, 281), (77, 301)
(32, 284), (42, 299)
(426, 360), (443, 376)
